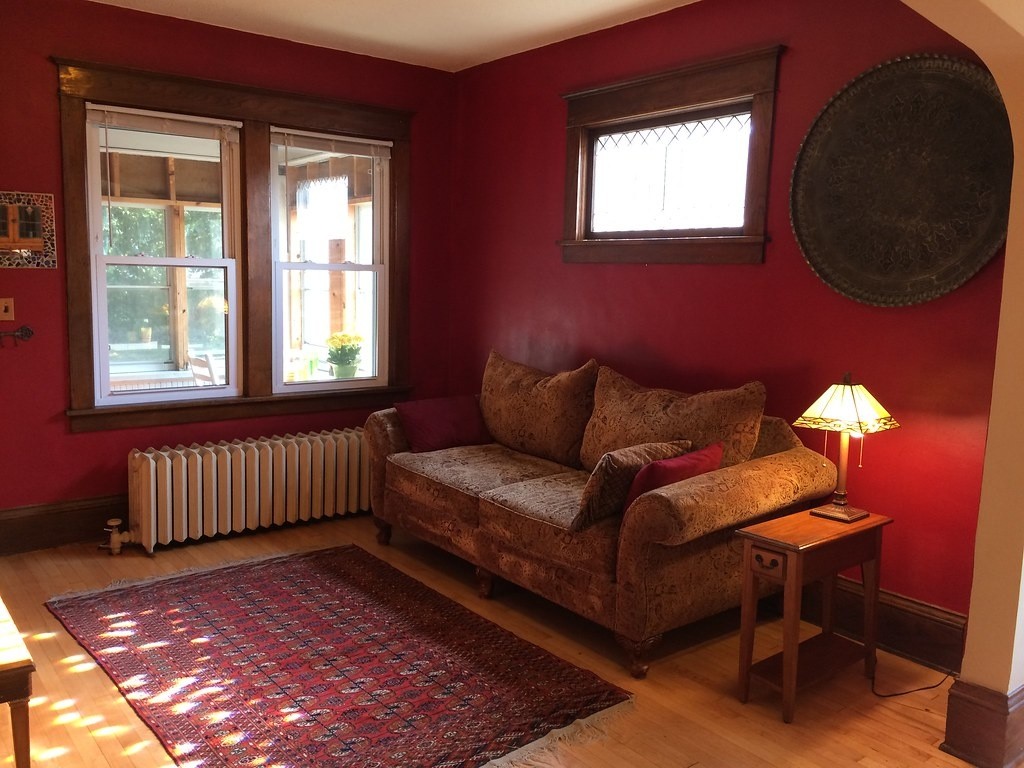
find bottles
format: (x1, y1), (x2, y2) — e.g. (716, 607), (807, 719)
(141, 319), (151, 342)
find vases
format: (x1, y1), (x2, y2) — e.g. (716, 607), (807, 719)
(331, 364), (357, 379)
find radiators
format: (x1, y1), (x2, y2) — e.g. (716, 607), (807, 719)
(104, 426), (371, 555)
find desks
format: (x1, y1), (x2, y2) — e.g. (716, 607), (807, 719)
(1, 591), (37, 768)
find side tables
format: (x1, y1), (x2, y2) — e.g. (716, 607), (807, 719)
(733, 503), (895, 723)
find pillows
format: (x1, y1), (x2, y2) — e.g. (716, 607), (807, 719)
(568, 440), (693, 533)
(391, 396), (494, 453)
(479, 348), (599, 470)
(580, 366), (766, 475)
(623, 442), (724, 517)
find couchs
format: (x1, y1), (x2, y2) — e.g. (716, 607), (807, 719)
(364, 402), (840, 681)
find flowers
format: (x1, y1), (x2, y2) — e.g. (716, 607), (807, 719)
(326, 330), (365, 366)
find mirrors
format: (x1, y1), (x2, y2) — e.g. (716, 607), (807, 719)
(0, 190), (57, 269)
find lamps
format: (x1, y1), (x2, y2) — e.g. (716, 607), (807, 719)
(792, 372), (901, 523)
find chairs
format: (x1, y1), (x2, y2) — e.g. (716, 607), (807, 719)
(187, 352), (216, 386)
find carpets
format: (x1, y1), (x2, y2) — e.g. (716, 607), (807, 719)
(41, 544), (638, 768)
